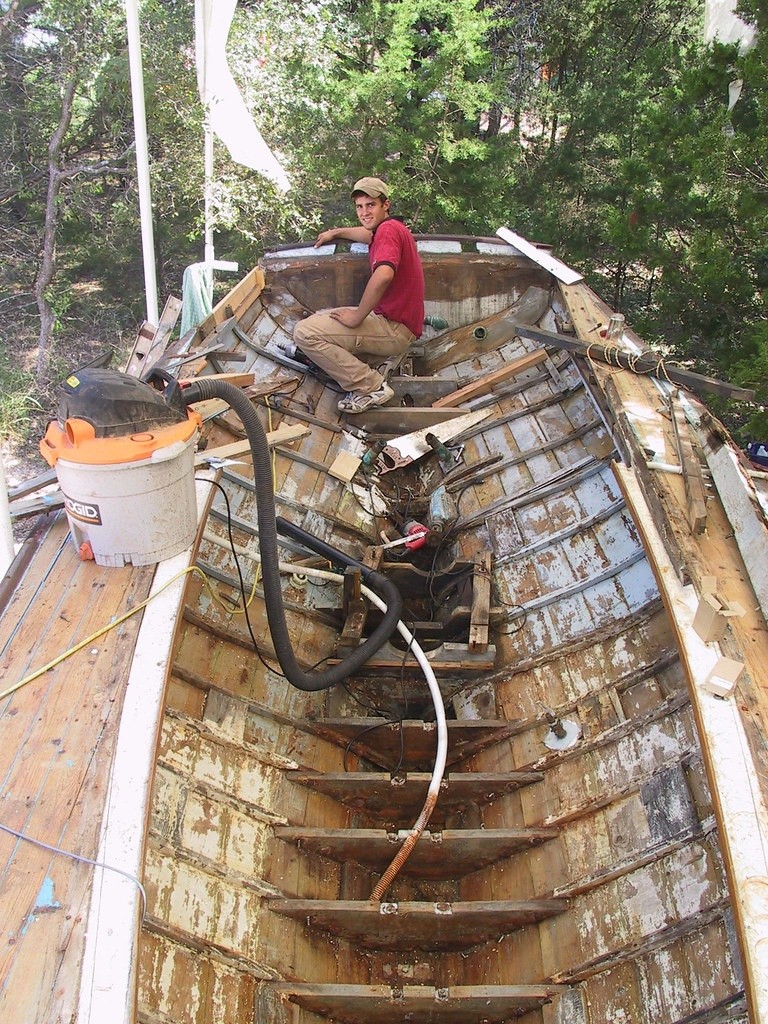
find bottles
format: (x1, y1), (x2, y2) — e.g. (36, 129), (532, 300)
(757, 446), (767, 457)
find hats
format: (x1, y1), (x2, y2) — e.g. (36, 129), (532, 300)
(350, 177), (389, 199)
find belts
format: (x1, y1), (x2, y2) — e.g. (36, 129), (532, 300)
(389, 321), (416, 343)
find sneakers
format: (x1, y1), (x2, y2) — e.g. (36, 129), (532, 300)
(338, 379), (395, 414)
(375, 352), (408, 382)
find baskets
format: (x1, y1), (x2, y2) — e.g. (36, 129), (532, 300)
(746, 441), (768, 467)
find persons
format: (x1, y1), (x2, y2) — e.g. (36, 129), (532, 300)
(293, 178), (424, 414)
